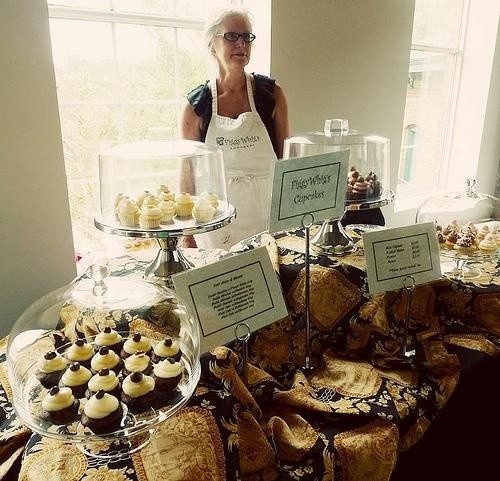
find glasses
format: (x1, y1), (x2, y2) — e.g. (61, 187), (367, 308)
(215, 31), (256, 43)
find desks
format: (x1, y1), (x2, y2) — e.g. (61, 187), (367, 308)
(0, 228), (500, 481)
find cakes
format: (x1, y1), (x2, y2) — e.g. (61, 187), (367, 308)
(152, 338), (182, 364)
(58, 362), (93, 399)
(345, 166), (381, 200)
(112, 185), (175, 229)
(173, 192), (195, 216)
(94, 326), (123, 356)
(124, 351), (153, 376)
(90, 346), (124, 374)
(35, 351), (71, 390)
(41, 386), (80, 425)
(192, 198), (216, 223)
(84, 368), (121, 400)
(198, 191), (219, 210)
(64, 339), (96, 370)
(80, 389), (123, 436)
(432, 220), (500, 255)
(122, 371), (156, 405)
(120, 332), (152, 359)
(152, 357), (183, 392)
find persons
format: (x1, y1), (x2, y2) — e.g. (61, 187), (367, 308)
(177, 7), (288, 249)
